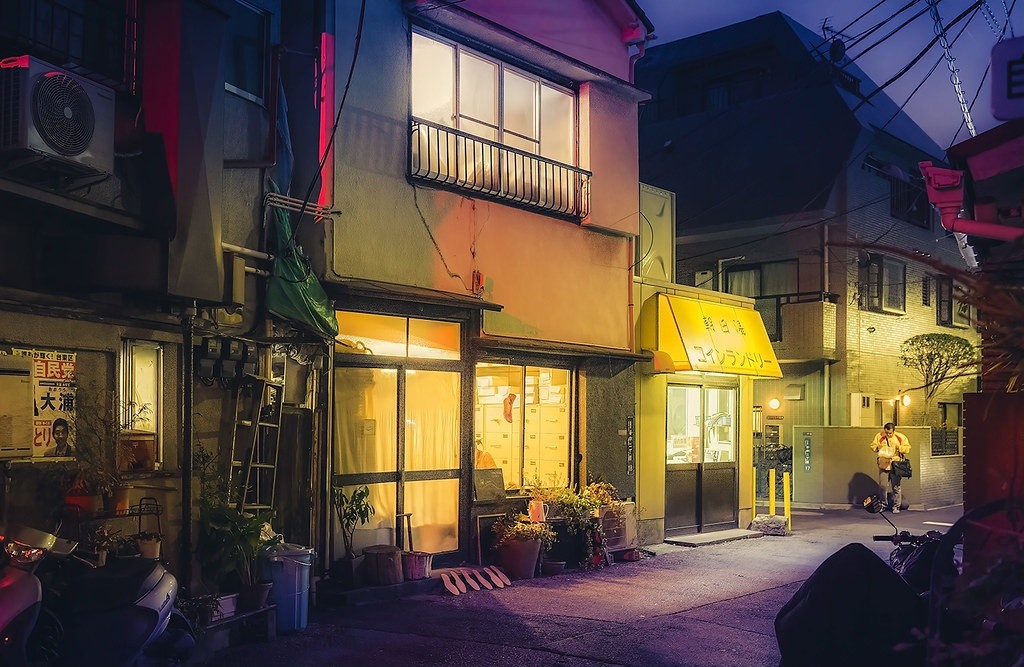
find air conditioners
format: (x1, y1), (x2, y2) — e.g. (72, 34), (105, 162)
(596, 502), (638, 553)
(0, 55), (117, 177)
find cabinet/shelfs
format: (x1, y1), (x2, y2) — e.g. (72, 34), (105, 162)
(63, 497), (164, 562)
(475, 405), (569, 489)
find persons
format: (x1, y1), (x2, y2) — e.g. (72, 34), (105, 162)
(44, 418), (75, 457)
(871, 422), (911, 513)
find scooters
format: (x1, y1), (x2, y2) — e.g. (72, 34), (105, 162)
(1, 502), (198, 667)
(862, 494), (943, 585)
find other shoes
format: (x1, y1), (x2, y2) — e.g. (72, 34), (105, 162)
(893, 506), (900, 513)
(880, 507), (885, 512)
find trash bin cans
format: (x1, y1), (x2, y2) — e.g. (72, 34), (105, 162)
(253, 542), (316, 631)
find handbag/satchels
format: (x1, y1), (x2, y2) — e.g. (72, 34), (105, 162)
(892, 453), (914, 477)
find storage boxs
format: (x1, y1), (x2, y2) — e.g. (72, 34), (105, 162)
(477, 367), (567, 405)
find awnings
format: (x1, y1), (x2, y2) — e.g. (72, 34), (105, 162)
(658, 293), (786, 380)
(330, 276), (505, 312)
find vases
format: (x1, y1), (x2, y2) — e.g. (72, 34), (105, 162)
(212, 592), (239, 623)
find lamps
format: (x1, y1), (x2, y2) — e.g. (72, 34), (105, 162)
(901, 394), (911, 407)
(768, 398), (781, 410)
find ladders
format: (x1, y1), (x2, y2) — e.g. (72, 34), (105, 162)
(225, 370), (284, 518)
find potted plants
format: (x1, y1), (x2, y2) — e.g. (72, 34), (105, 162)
(56, 370), (148, 515)
(495, 514), (556, 582)
(203, 498), (279, 608)
(83, 526), (121, 566)
(332, 485), (375, 588)
(133, 531), (168, 560)
(181, 588), (225, 640)
